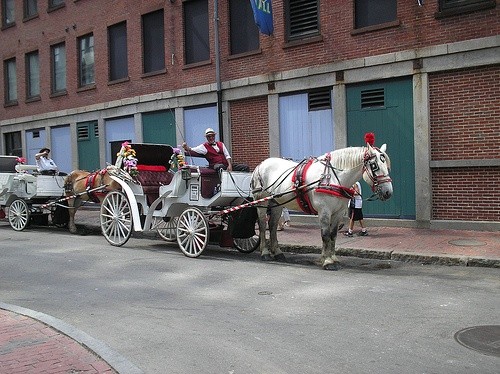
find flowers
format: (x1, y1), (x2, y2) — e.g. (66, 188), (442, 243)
(116, 141), (139, 177)
(168, 146), (188, 172)
(16, 157), (26, 164)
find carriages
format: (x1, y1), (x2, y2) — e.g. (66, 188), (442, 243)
(98, 131), (394, 272)
(0, 151), (122, 235)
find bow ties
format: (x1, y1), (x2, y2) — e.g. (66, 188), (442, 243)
(209, 143), (216, 146)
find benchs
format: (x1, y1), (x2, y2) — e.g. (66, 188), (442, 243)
(129, 170), (173, 209)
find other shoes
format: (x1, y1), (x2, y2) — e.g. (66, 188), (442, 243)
(343, 231), (354, 237)
(356, 230), (368, 236)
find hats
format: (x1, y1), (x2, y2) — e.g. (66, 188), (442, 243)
(203, 128), (216, 136)
(39, 148), (50, 154)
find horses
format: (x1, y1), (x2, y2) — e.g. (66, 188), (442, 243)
(251, 144), (393, 272)
(63, 161), (121, 234)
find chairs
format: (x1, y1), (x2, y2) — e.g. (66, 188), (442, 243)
(178, 150), (220, 198)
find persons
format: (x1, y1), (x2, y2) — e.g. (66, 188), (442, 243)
(267, 205), (290, 231)
(182, 128), (250, 184)
(35, 147), (68, 176)
(344, 181), (368, 237)
